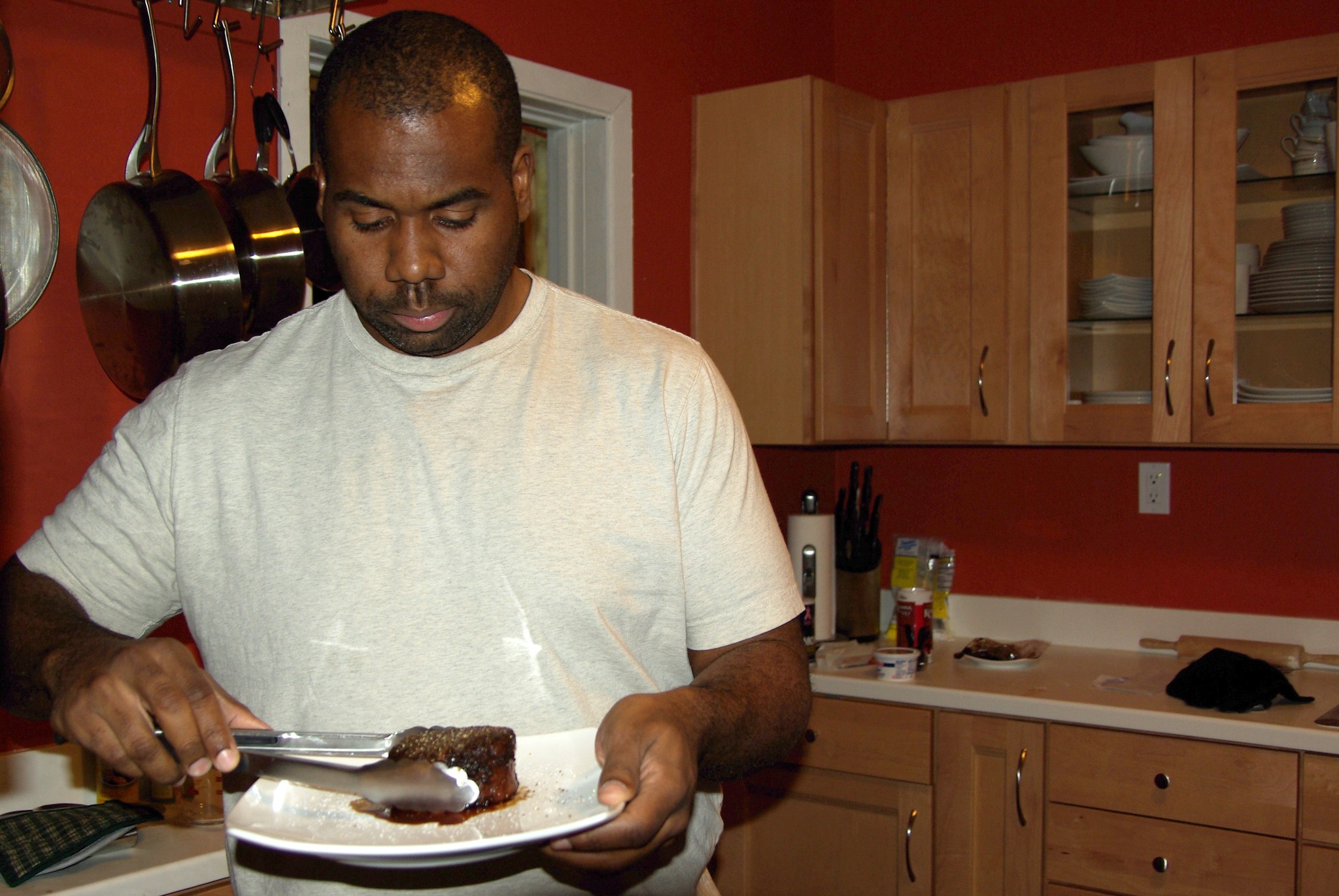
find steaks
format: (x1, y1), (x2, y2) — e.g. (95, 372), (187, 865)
(388, 725), (520, 807)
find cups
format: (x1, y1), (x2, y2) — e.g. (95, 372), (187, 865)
(1234, 242), (1261, 315)
(874, 647), (919, 683)
(1281, 90), (1337, 175)
(897, 588), (933, 668)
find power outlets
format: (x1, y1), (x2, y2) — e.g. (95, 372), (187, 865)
(1138, 462), (1171, 515)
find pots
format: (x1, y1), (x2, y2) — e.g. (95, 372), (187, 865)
(75, 0), (345, 401)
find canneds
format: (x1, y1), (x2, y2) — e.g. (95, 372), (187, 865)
(895, 586), (933, 665)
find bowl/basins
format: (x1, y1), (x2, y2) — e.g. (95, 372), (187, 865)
(1078, 114), (1156, 175)
(1236, 129), (1246, 152)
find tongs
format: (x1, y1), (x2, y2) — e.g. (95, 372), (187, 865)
(157, 726), (481, 816)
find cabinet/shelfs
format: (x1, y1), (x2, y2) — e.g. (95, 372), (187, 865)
(690, 74), (886, 448)
(706, 693), (935, 896)
(932, 707), (1045, 896)
(1026, 27), (1339, 449)
(883, 79), (1029, 446)
(1041, 719), (1339, 896)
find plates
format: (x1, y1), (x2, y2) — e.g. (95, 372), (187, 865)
(1247, 198), (1334, 312)
(1236, 164), (1271, 180)
(1067, 174), (1154, 198)
(225, 729), (627, 869)
(962, 636), (1044, 670)
(1077, 273), (1154, 320)
(1235, 383), (1336, 404)
(1083, 389), (1154, 404)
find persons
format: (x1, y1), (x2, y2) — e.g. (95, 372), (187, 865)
(0, 9), (809, 896)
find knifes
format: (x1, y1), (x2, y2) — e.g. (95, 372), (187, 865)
(834, 462), (886, 575)
